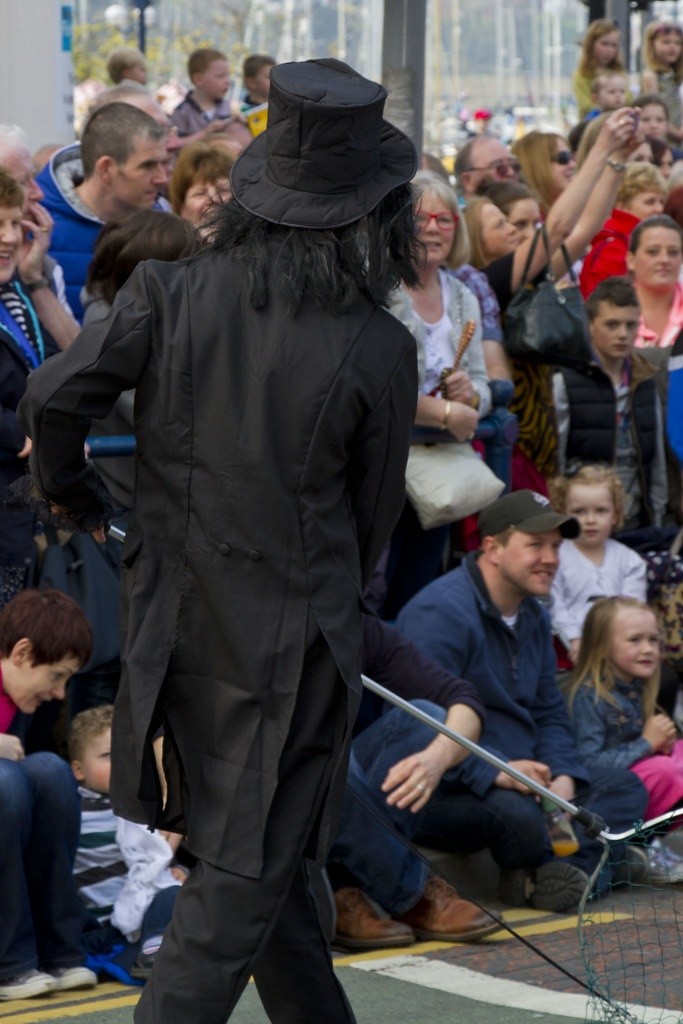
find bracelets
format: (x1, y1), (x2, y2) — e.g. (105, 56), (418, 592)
(442, 399), (450, 432)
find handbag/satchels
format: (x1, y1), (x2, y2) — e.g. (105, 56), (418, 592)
(642, 526), (683, 659)
(43, 522), (123, 671)
(502, 221), (592, 367)
(405, 440), (504, 529)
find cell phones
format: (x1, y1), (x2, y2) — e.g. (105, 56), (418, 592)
(626, 111), (640, 133)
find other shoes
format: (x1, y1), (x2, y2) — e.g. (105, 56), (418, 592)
(131, 929), (168, 976)
(643, 839), (683, 883)
(496, 861), (590, 912)
(613, 844), (646, 885)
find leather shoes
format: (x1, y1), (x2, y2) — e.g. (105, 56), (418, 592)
(330, 886), (414, 953)
(395, 871), (507, 942)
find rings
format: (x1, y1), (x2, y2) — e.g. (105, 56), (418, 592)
(418, 785), (424, 790)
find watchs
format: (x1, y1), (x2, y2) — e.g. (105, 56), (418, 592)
(606, 160), (627, 173)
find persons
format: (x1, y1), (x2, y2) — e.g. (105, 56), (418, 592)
(1, 0), (683, 1024)
(13, 56), (426, 1023)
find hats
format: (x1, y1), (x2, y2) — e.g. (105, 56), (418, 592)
(475, 490), (580, 540)
(232, 58), (419, 229)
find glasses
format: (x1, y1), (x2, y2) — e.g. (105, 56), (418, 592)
(414, 208), (458, 231)
(460, 162), (522, 176)
(550, 150), (576, 165)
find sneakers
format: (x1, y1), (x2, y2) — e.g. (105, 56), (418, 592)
(0, 966), (96, 999)
